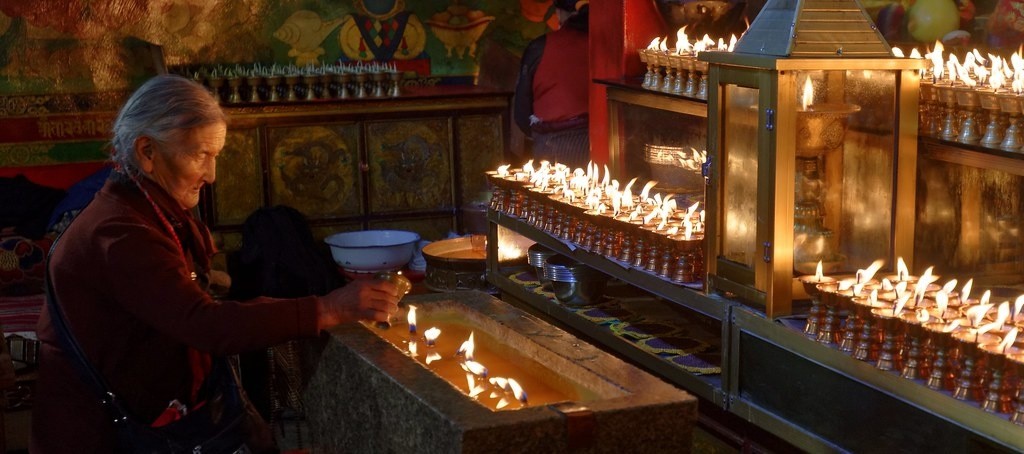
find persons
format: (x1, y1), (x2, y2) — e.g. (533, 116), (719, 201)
(512, 3), (590, 167)
(34, 74), (399, 454)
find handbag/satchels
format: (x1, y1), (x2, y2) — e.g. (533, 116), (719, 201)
(119, 383), (254, 454)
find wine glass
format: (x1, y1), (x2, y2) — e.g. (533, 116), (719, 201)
(184, 66), (404, 105)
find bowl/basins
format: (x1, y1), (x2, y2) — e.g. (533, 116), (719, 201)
(527, 243), (569, 291)
(422, 234), (500, 294)
(322, 229), (421, 273)
(543, 254), (609, 307)
(631, 128), (707, 194)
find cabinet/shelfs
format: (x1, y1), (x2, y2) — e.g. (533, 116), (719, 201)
(485, 76), (1024, 454)
